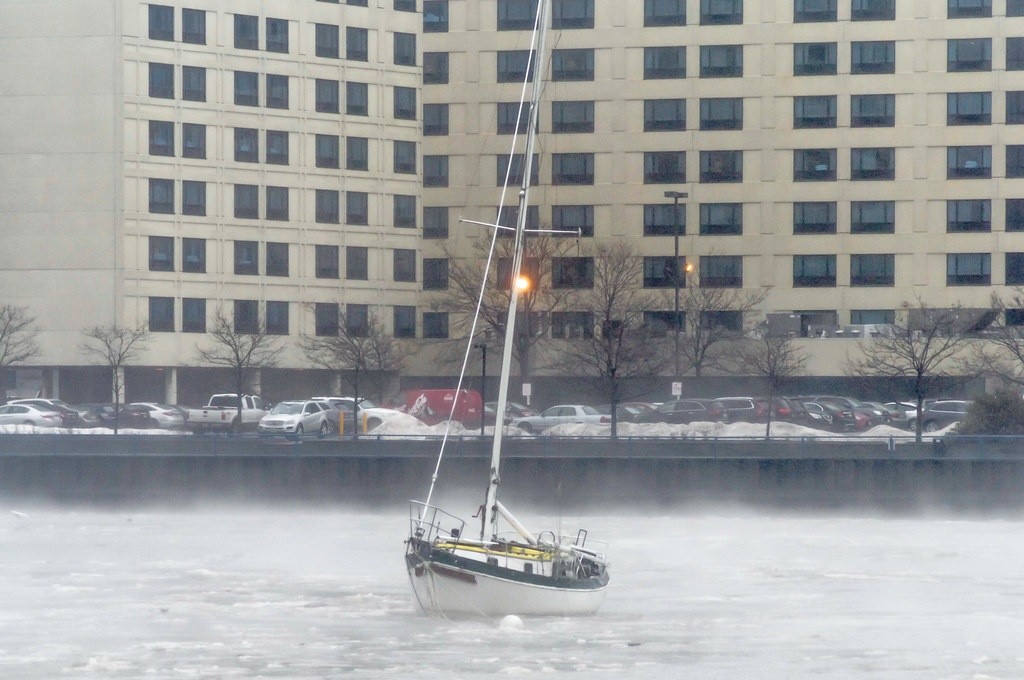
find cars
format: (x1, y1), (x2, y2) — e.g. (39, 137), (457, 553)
(0, 396), (185, 431)
(482, 396), (976, 435)
(257, 397), (402, 441)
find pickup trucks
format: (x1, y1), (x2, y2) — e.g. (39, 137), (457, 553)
(187, 393), (274, 436)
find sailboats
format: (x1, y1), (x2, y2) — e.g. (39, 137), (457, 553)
(401, 0), (612, 622)
